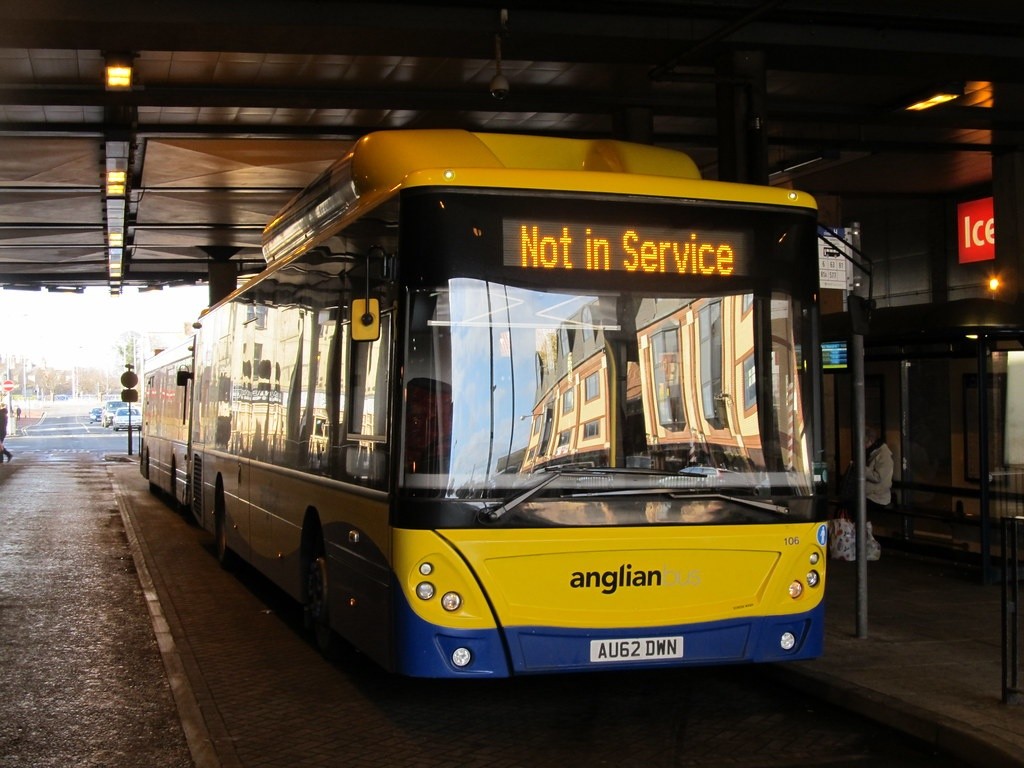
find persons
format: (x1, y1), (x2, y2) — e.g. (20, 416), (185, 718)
(843, 419), (894, 520)
(0, 408), (13, 463)
(17, 407), (21, 419)
(828, 504), (881, 617)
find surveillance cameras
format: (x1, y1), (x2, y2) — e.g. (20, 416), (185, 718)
(490, 75), (510, 100)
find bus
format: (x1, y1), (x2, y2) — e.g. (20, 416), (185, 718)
(138, 128), (876, 682)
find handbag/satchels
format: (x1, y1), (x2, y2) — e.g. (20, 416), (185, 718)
(825, 503), (881, 561)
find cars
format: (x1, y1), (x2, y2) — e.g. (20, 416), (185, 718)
(88, 407), (103, 424)
(112, 407), (142, 431)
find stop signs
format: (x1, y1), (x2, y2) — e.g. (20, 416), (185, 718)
(3, 380), (14, 391)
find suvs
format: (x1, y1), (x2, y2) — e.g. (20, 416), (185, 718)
(101, 400), (128, 428)
